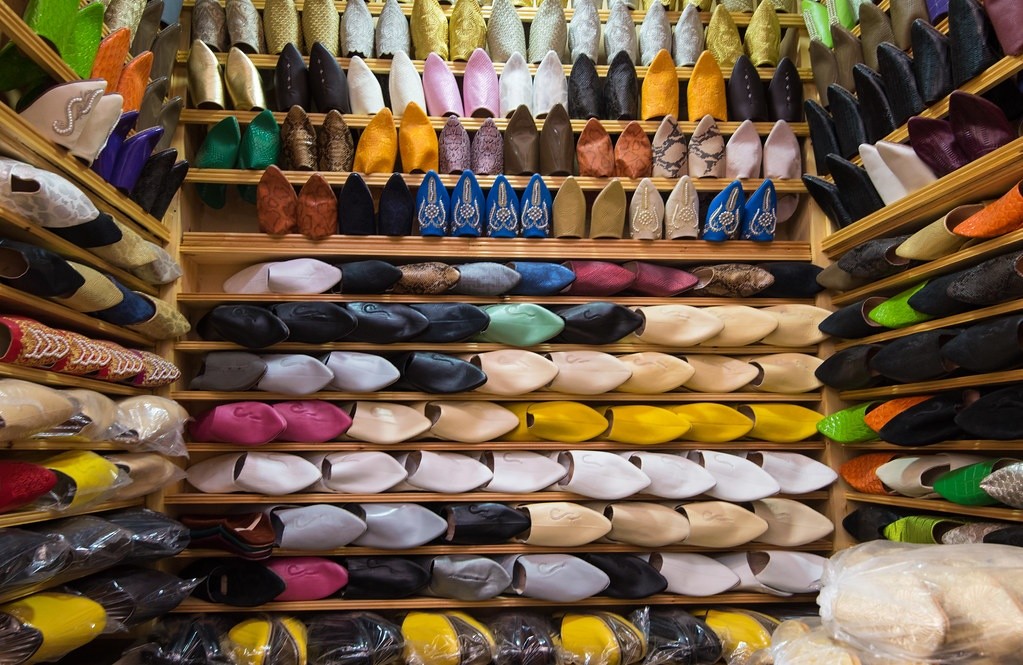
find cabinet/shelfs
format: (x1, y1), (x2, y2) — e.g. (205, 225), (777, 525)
(0, 0), (1023, 652)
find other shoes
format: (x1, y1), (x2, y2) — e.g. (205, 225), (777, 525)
(0, 0), (1023, 665)
